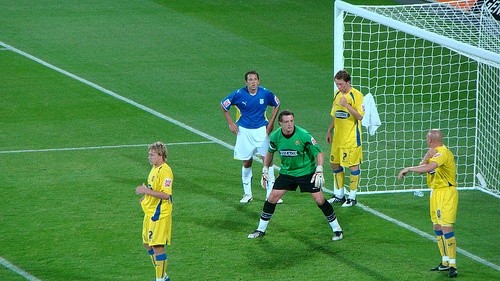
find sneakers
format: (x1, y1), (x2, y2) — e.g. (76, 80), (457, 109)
(342, 198), (356, 206)
(327, 195), (345, 203)
(248, 230), (265, 239)
(332, 231), (344, 241)
(240, 194), (254, 202)
(265, 197), (282, 203)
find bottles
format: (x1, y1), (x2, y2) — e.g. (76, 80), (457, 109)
(412, 191), (424, 197)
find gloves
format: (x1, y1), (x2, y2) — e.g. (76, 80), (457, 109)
(261, 167), (271, 190)
(310, 166), (325, 189)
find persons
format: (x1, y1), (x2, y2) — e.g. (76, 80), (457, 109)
(247, 109), (343, 241)
(134, 140), (174, 281)
(397, 128), (459, 278)
(326, 69), (366, 208)
(220, 71), (284, 204)
